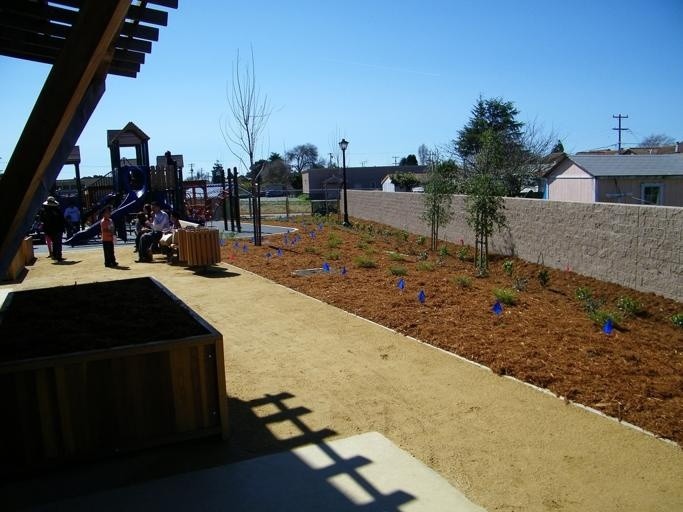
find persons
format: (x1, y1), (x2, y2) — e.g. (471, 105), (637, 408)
(100, 207), (118, 267)
(133, 202), (181, 263)
(64, 201), (81, 240)
(39, 196), (65, 260)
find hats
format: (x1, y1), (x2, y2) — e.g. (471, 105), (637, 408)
(42, 196), (60, 206)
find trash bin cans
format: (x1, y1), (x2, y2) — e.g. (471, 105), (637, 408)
(179, 228), (220, 267)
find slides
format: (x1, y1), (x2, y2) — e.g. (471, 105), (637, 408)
(62, 182), (147, 246)
(158, 191), (205, 227)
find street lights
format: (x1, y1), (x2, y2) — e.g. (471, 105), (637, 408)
(338, 139), (353, 228)
